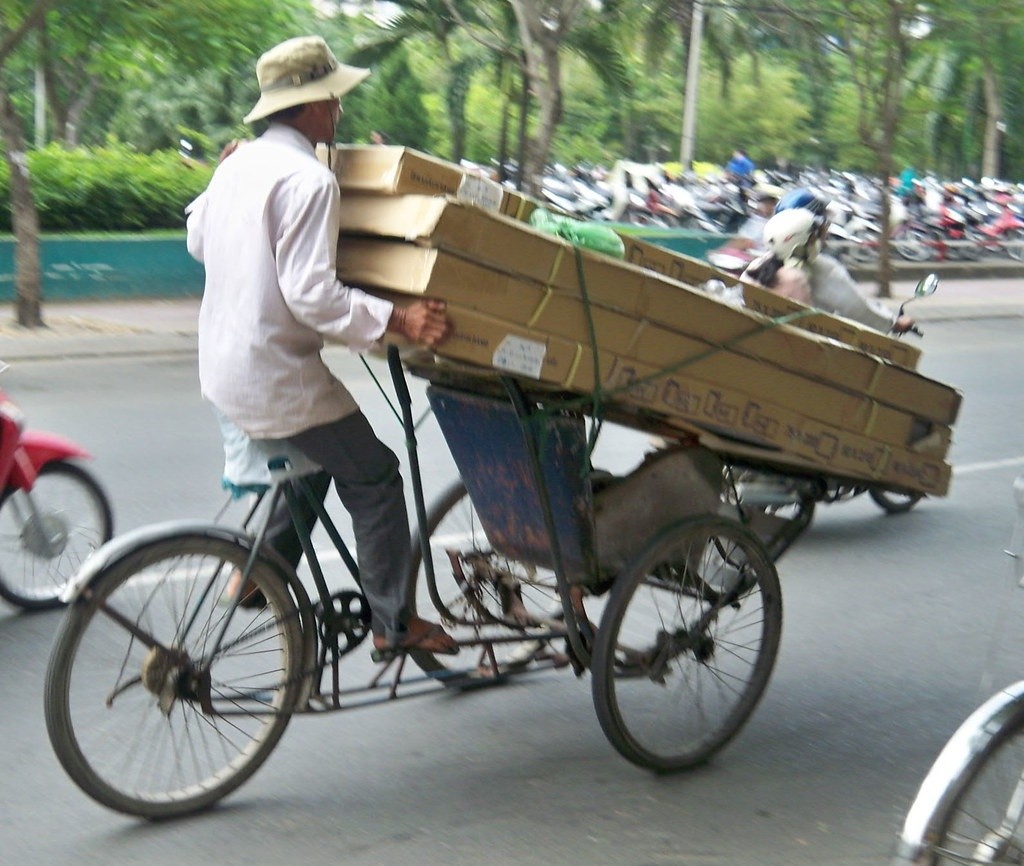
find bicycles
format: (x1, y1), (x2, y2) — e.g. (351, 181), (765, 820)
(895, 680), (1024, 865)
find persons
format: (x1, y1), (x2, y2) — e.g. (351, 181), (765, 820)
(183, 36), (466, 662)
(711, 146), (916, 340)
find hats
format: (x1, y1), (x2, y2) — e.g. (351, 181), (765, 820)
(242, 35), (371, 123)
(745, 181), (782, 200)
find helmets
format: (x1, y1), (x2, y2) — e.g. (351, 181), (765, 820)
(773, 187), (835, 228)
(762, 208), (824, 261)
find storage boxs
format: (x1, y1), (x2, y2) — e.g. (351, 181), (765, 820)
(337, 237), (953, 460)
(327, 147), (923, 371)
(371, 291), (952, 498)
(337, 189), (966, 429)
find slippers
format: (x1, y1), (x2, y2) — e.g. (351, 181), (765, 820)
(369, 619), (458, 662)
(221, 589), (266, 609)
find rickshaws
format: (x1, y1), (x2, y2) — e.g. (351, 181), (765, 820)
(44, 344), (807, 820)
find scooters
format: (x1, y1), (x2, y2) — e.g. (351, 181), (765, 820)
(0, 363), (112, 609)
(492, 155), (1024, 262)
(723, 275), (939, 532)
